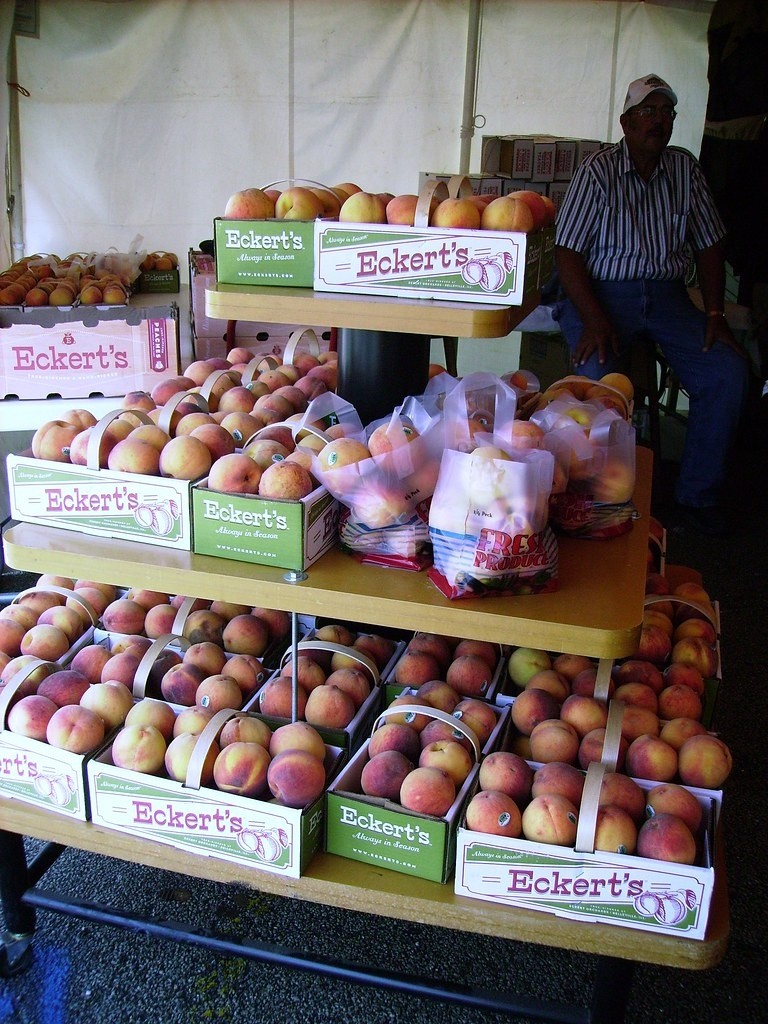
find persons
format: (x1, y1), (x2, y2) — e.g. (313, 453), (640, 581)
(552, 76), (752, 536)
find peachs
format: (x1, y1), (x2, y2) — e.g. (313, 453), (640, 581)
(32, 345), (637, 597)
(0, 252), (179, 306)
(360, 517), (733, 868)
(0, 574), (391, 811)
(224, 183), (557, 235)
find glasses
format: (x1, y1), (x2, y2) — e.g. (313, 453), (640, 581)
(628, 107), (677, 123)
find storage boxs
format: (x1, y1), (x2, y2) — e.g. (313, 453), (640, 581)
(537, 225), (558, 291)
(189, 476), (347, 571)
(481, 133), (615, 182)
(450, 174), (480, 196)
(549, 181), (572, 212)
(503, 179), (526, 196)
(0, 585), (726, 942)
(134, 251), (181, 294)
(473, 173), (502, 195)
(418, 172), (452, 196)
(312, 179), (543, 307)
(77, 280), (130, 308)
(0, 281), (26, 312)
(6, 408), (243, 552)
(24, 282), (80, 313)
(526, 182), (546, 195)
(213, 178), (343, 288)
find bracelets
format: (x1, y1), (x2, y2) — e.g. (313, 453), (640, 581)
(708, 308), (727, 319)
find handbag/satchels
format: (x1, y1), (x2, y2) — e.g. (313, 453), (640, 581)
(292, 369), (640, 602)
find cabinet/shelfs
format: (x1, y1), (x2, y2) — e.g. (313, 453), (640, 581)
(0, 293), (732, 1024)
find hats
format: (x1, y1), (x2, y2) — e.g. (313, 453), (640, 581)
(622, 73), (678, 114)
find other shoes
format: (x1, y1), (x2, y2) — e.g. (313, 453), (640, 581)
(675, 500), (728, 537)
